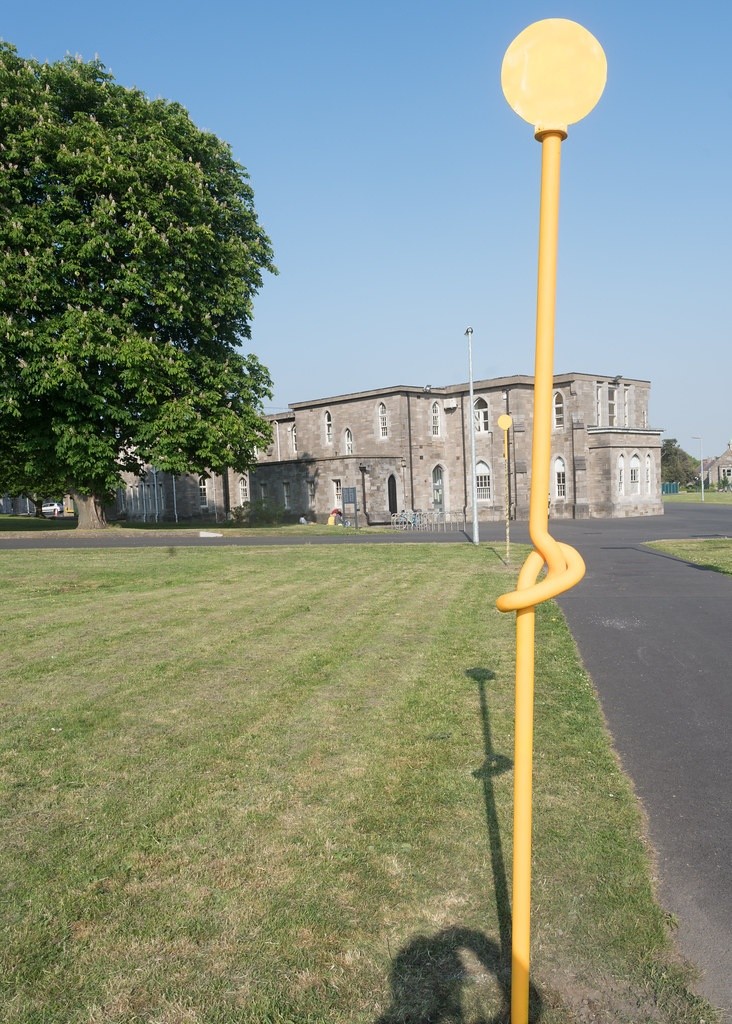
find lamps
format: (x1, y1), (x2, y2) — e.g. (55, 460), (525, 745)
(423, 384), (432, 392)
(614, 374), (623, 382)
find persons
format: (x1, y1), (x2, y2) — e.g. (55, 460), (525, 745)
(300, 514), (308, 524)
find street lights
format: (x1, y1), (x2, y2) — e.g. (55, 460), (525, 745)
(464, 326), (480, 545)
(692, 436), (704, 502)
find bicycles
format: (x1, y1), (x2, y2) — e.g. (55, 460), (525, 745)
(394, 508), (428, 530)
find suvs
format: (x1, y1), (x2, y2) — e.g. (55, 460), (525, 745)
(42, 503), (63, 514)
